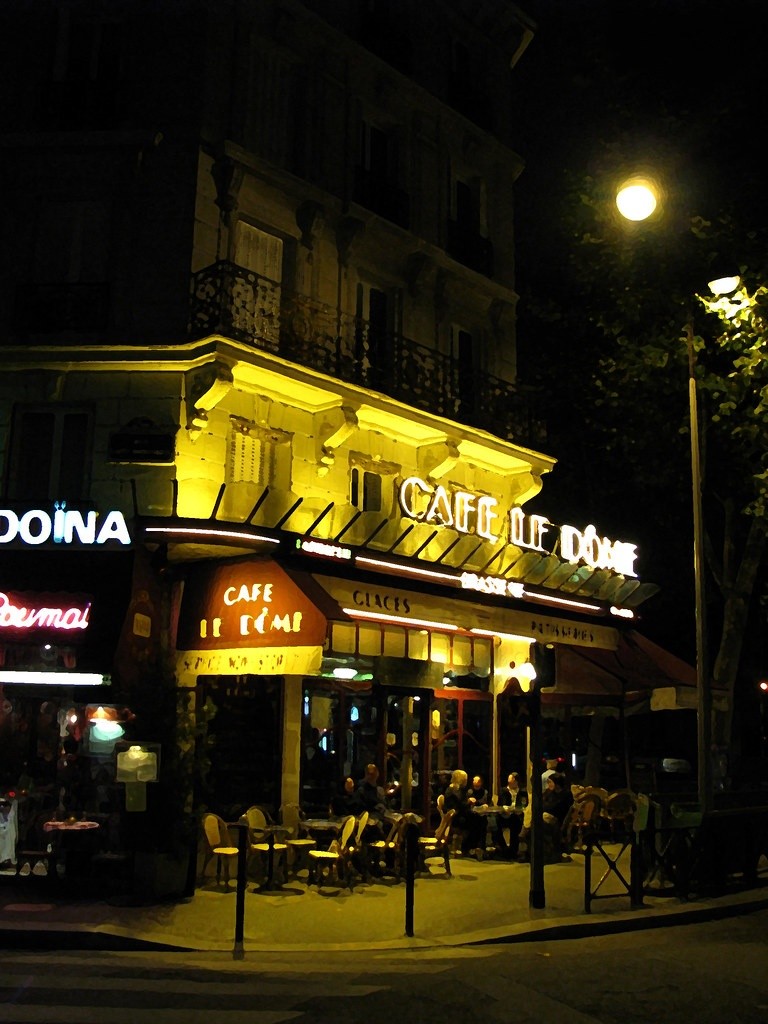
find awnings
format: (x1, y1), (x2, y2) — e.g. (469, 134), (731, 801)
(550, 635), (700, 694)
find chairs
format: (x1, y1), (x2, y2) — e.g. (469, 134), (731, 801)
(16, 809), (55, 878)
(201, 786), (632, 892)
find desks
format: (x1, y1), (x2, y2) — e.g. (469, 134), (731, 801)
(475, 804), (523, 862)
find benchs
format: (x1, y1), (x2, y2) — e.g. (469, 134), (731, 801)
(300, 818), (378, 879)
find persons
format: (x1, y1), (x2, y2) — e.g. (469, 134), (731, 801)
(444, 769), (572, 860)
(336, 761), (398, 883)
(23, 735), (59, 850)
(301, 728), (332, 785)
